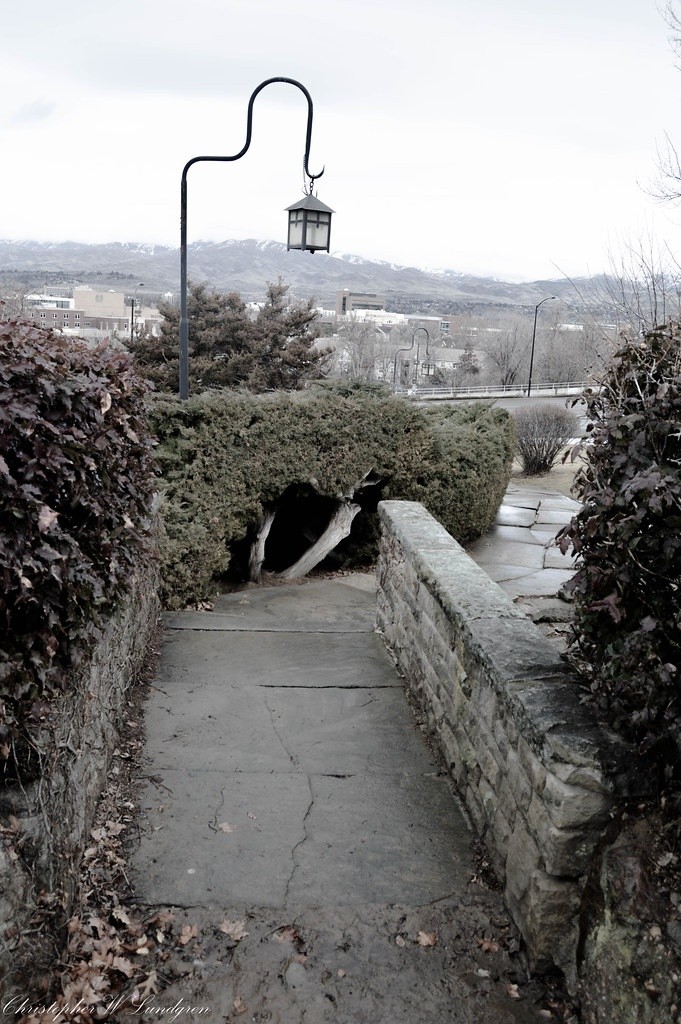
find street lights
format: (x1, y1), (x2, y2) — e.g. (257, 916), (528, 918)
(391, 327), (431, 394)
(527, 296), (559, 396)
(130, 282), (145, 347)
(177, 77), (337, 403)
